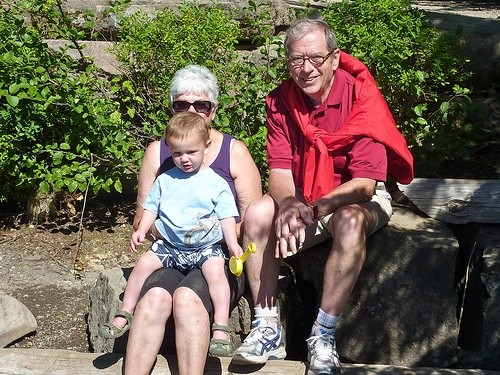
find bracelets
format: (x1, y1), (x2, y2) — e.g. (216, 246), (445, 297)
(311, 205), (319, 221)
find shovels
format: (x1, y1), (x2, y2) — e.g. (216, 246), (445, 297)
(227, 241), (258, 277)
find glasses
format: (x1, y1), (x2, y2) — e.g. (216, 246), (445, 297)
(169, 98), (216, 114)
(286, 47), (337, 67)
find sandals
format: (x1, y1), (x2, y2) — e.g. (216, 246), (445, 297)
(98, 310), (134, 338)
(209, 323), (235, 357)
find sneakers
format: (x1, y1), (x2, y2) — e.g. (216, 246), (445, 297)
(304, 324), (343, 374)
(233, 317), (288, 366)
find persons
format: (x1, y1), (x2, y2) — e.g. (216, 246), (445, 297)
(120, 63), (264, 375)
(231, 18), (415, 373)
(97, 111), (244, 356)
